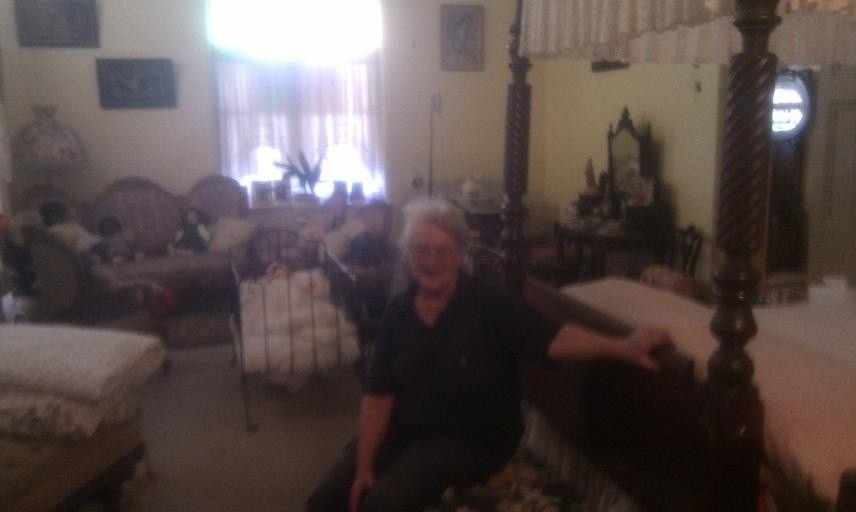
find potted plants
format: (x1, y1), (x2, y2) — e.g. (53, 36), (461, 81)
(274, 149), (327, 206)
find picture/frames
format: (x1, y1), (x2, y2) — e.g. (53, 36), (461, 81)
(439, 3), (486, 73)
(93, 57), (177, 111)
(14, 0), (100, 49)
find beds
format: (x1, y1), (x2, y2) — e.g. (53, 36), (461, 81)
(225, 226), (363, 434)
(516, 257), (854, 511)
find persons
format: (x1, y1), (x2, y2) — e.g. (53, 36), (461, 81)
(40, 201), (177, 308)
(303, 198), (670, 512)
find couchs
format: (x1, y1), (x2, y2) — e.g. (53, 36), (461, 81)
(16, 174), (248, 297)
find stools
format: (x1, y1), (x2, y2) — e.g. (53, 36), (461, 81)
(0, 389), (142, 510)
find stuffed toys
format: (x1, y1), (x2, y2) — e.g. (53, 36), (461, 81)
(172, 207), (209, 255)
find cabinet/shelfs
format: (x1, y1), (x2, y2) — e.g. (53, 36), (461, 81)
(554, 216), (685, 285)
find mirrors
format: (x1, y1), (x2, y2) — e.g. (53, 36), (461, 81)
(606, 105), (653, 203)
